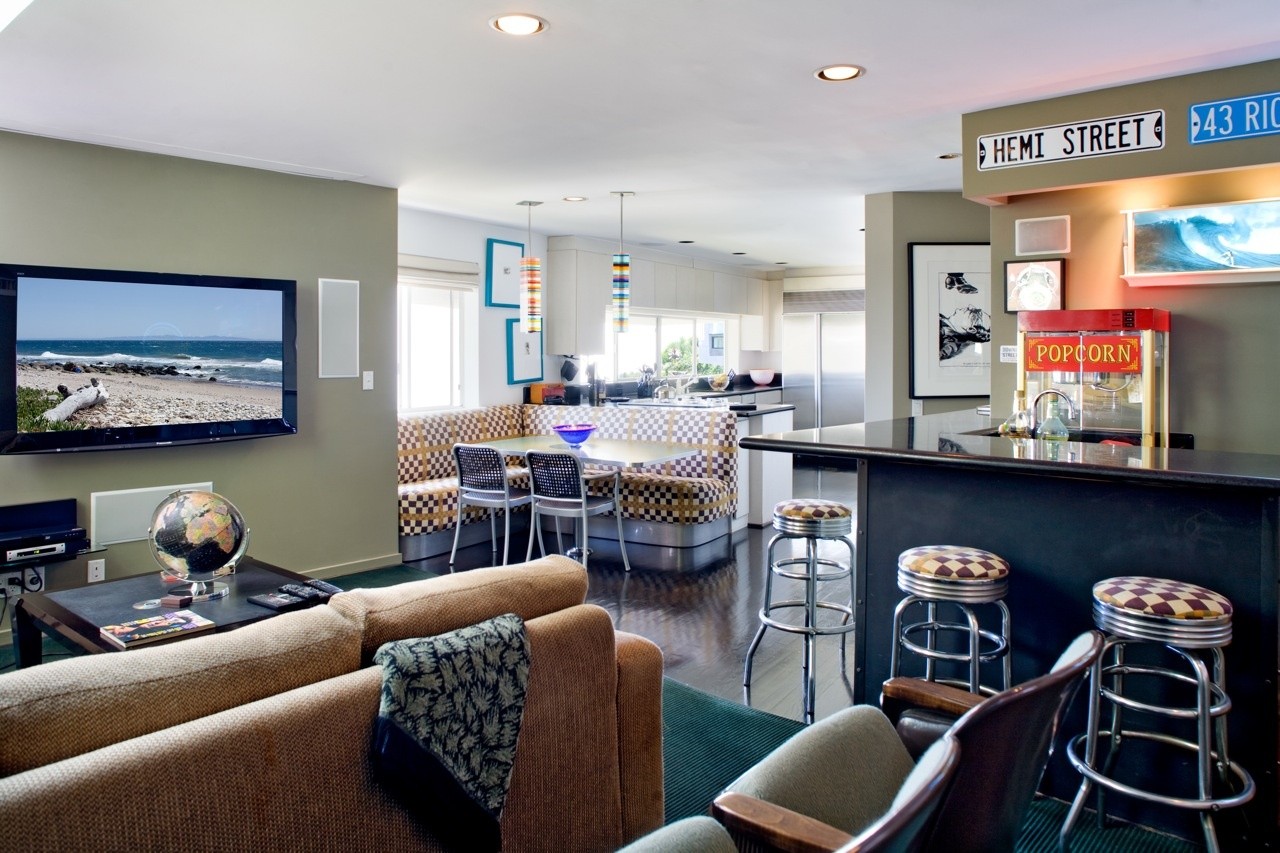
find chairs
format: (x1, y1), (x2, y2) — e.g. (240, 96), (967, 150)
(713, 628), (1105, 853)
(448, 443), (545, 566)
(525, 450), (632, 571)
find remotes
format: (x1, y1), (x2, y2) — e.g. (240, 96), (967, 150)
(247, 578), (296, 612)
(300, 585), (330, 605)
(279, 583), (319, 607)
(303, 578), (344, 595)
(269, 590), (306, 610)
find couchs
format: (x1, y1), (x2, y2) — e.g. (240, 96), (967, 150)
(396, 403), (737, 563)
(2, 555), (663, 853)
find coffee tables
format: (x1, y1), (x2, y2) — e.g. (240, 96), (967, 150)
(9, 556), (343, 669)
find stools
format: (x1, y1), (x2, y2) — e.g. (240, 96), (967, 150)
(1059, 576), (1257, 853)
(742, 499), (856, 725)
(879, 545), (1011, 713)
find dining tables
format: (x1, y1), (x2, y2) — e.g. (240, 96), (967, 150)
(474, 436), (698, 553)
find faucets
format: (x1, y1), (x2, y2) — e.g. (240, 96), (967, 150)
(668, 375), (700, 397)
(1028, 389), (1076, 441)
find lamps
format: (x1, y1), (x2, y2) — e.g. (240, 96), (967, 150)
(517, 200), (542, 333)
(609, 191), (635, 328)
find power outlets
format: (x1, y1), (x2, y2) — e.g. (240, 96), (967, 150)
(0, 572), (22, 598)
(24, 566), (45, 593)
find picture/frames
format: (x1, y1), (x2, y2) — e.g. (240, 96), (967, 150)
(505, 318), (544, 385)
(1004, 257), (1066, 313)
(484, 238), (525, 308)
(908, 240), (991, 397)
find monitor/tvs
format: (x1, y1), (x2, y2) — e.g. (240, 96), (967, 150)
(1, 264), (298, 456)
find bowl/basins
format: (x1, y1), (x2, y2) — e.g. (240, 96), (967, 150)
(709, 377), (729, 391)
(749, 369), (775, 385)
(552, 424), (597, 447)
